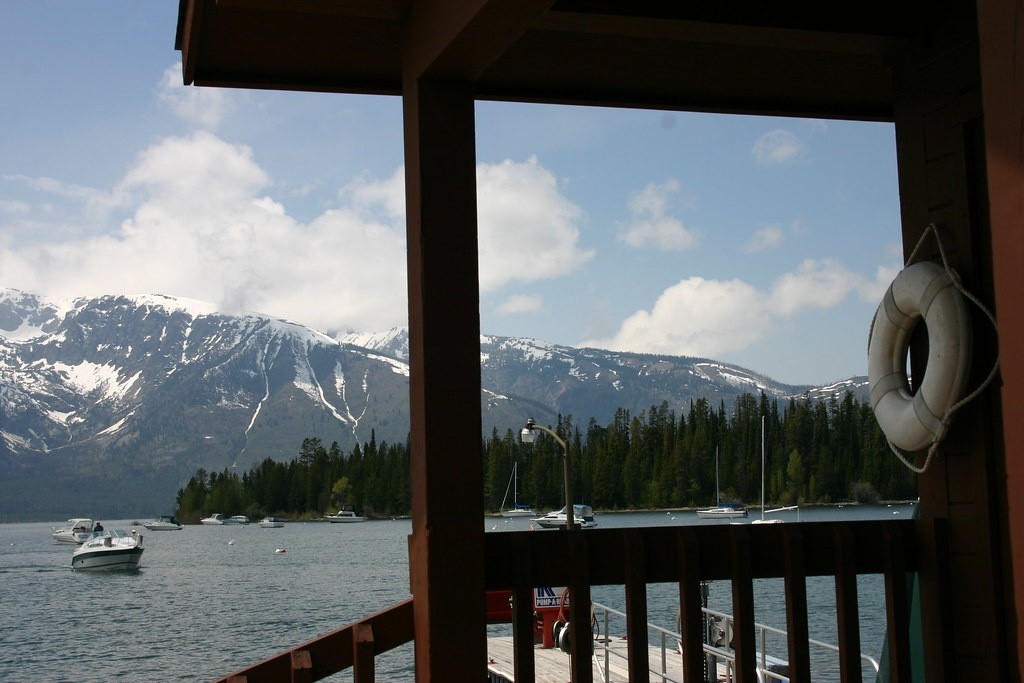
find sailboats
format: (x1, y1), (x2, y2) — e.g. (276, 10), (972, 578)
(694, 445), (748, 518)
(498, 460), (535, 516)
(727, 413), (800, 524)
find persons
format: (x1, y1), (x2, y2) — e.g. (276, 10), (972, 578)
(94, 522), (103, 538)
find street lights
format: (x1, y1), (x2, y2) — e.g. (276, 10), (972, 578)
(521, 418), (576, 529)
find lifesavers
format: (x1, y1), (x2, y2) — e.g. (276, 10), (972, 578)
(866, 257), (974, 452)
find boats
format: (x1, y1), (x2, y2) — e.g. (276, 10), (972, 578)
(71, 528), (144, 569)
(225, 516), (250, 525)
(528, 505), (596, 529)
(324, 511), (365, 522)
(258, 516), (284, 528)
(201, 514), (225, 525)
(144, 514), (184, 531)
(52, 518), (96, 544)
